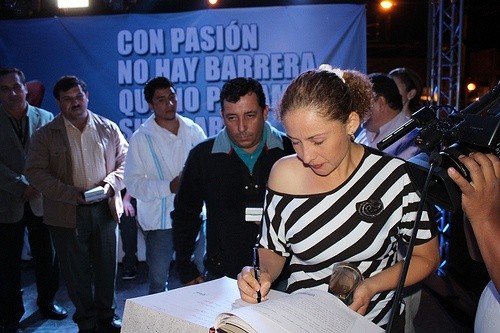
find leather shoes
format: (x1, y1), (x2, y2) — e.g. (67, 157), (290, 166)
(4, 321), (30, 333)
(39, 304), (68, 319)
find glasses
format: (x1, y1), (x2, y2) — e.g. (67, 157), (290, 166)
(0, 84), (25, 92)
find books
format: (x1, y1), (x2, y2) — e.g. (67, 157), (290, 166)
(120, 275), (386, 333)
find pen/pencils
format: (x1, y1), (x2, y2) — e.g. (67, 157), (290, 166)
(252, 247), (261, 302)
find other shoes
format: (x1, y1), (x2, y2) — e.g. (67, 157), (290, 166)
(120, 258), (138, 280)
(79, 320), (120, 333)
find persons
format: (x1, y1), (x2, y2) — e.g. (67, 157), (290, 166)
(122, 75), (208, 295)
(0, 67), (69, 333)
(237, 63), (441, 333)
(447, 151), (500, 333)
(353, 68), (437, 159)
(170, 76), (297, 287)
(22, 75), (128, 333)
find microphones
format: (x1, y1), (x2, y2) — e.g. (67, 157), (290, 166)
(377, 105), (437, 152)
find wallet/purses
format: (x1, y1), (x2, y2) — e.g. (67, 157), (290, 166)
(327, 262), (364, 307)
